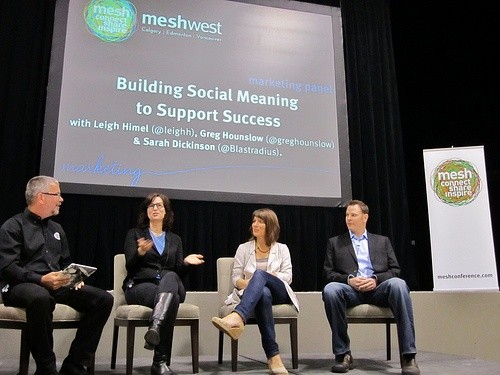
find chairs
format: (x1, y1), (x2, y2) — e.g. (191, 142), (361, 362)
(109, 254), (201, 375)
(217, 257), (299, 372)
(347, 305), (396, 362)
(0, 302), (79, 375)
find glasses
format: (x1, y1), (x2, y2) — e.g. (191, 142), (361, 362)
(37, 192), (61, 199)
(148, 203), (164, 209)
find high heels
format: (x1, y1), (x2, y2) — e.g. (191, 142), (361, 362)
(268, 355), (288, 375)
(211, 317), (244, 340)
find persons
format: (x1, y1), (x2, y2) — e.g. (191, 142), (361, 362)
(0, 176), (114, 375)
(322, 200), (420, 375)
(121, 192), (205, 375)
(212, 209), (300, 375)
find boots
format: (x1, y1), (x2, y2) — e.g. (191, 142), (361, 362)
(143, 292), (174, 350)
(150, 324), (175, 375)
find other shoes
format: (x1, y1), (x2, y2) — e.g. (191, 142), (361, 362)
(59, 361), (87, 375)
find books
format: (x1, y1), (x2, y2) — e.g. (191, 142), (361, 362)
(61, 263), (97, 289)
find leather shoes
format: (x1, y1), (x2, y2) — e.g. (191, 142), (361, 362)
(332, 350), (353, 372)
(401, 353), (419, 375)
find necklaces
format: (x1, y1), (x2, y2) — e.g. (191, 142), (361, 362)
(256, 244), (270, 253)
